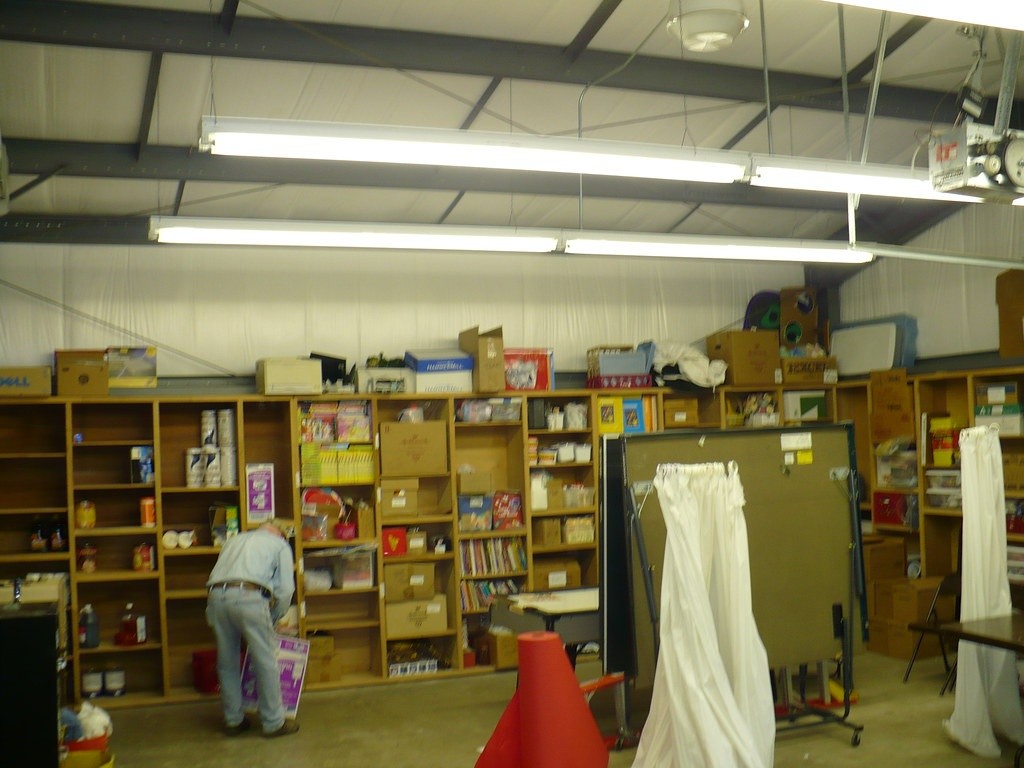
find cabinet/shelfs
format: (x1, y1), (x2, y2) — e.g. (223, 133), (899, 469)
(0, 360), (1024, 713)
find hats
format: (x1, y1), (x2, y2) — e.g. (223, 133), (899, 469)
(259, 518), (288, 540)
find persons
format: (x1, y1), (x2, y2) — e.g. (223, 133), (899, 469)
(204, 518), (300, 738)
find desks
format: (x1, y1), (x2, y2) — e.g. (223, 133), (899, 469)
(0, 573), (67, 768)
(507, 587), (600, 690)
(939, 613), (1024, 653)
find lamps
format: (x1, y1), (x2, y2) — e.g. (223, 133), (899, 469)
(148, 0), (566, 252)
(751, 0), (1024, 206)
(198, 0), (750, 183)
(564, 0), (878, 263)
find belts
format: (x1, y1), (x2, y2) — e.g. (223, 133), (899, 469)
(212, 582), (261, 591)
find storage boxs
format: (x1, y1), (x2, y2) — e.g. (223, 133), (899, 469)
(867, 611), (943, 660)
(931, 439), (962, 467)
(382, 526), (408, 557)
(354, 366), (415, 394)
(1002, 449), (1024, 491)
(484, 393), (523, 422)
(459, 495), (496, 534)
(624, 395), (644, 434)
(535, 516), (562, 544)
(403, 346), (473, 392)
(549, 476), (565, 509)
(927, 486), (963, 507)
(334, 414), (372, 445)
(877, 575), (957, 618)
(785, 387), (828, 421)
(925, 468), (961, 487)
(456, 322), (510, 392)
(871, 443), (917, 488)
(107, 347), (157, 389)
(330, 553), (374, 589)
(705, 328), (782, 384)
(383, 558), (436, 605)
(503, 344), (558, 391)
(975, 380), (1020, 406)
(407, 529), (428, 554)
(55, 350), (108, 397)
(255, 357), (325, 398)
(384, 590), (450, 637)
(743, 412), (782, 427)
(302, 514), (328, 541)
(0, 366), (54, 398)
(664, 393), (697, 428)
(458, 465), (494, 498)
(863, 529), (907, 621)
(305, 650), (343, 684)
(974, 406), (1024, 438)
(211, 527), (239, 547)
(380, 415), (448, 479)
(870, 491), (905, 525)
(529, 395), (546, 429)
(785, 352), (846, 382)
(597, 395), (623, 435)
(381, 475), (424, 518)
(929, 416), (968, 437)
(531, 483), (548, 510)
(207, 502), (238, 528)
(309, 628), (335, 657)
(487, 621), (516, 672)
(347, 504), (377, 538)
(535, 554), (584, 591)
(309, 351), (349, 380)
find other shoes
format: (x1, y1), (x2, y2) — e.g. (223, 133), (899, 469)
(225, 716), (258, 736)
(262, 718), (300, 737)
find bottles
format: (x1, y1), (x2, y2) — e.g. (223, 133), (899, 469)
(75, 499), (96, 529)
(186, 447), (203, 488)
(132, 538), (155, 572)
(50, 513), (69, 553)
(217, 409), (236, 446)
(201, 446), (222, 488)
(140, 496), (156, 528)
(201, 410), (217, 448)
(81, 667), (102, 697)
(103, 665), (127, 698)
(31, 515), (47, 553)
(219, 447), (237, 487)
(77, 542), (98, 573)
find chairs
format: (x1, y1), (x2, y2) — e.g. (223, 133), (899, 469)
(903, 574), (961, 696)
